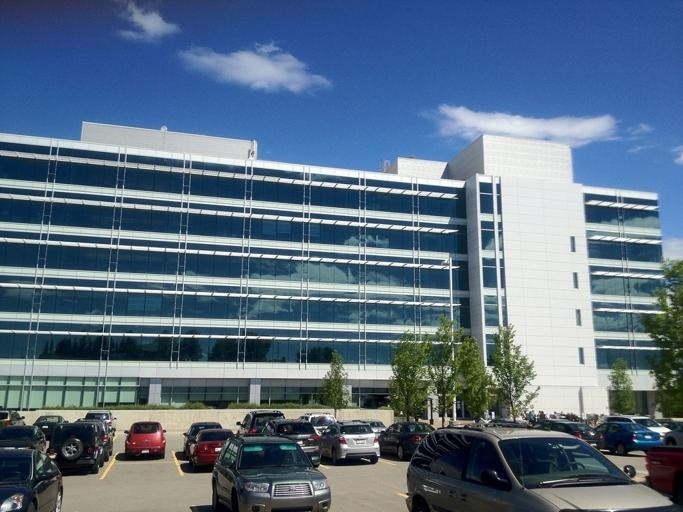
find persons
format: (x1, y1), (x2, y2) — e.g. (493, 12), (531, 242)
(521, 409), (578, 423)
